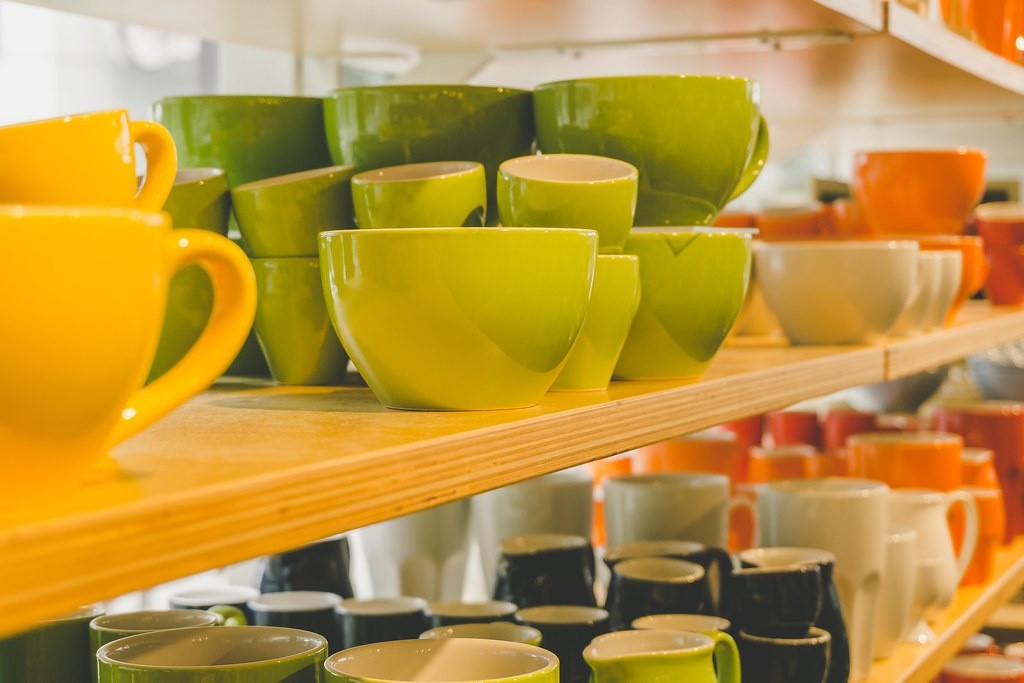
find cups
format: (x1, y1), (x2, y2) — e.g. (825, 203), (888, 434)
(0, 73), (1024, 683)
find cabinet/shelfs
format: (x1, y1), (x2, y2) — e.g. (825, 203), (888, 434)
(1, 0), (1024, 682)
